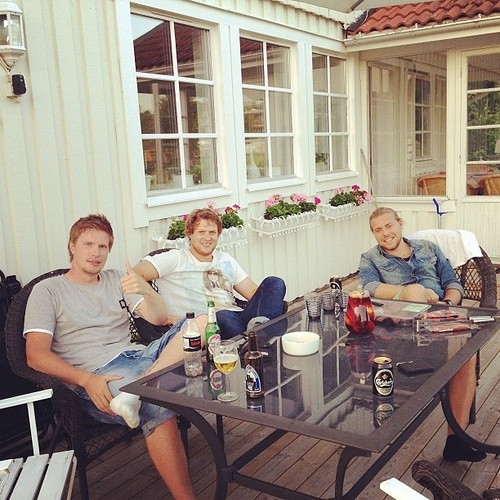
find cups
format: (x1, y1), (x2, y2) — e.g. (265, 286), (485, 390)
(305, 289), (350, 321)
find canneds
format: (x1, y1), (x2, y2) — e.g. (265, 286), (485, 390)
(373, 357), (393, 397)
(329, 277), (342, 289)
(373, 395), (394, 428)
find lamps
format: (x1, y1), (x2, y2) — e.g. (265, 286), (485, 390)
(0, 0), (27, 102)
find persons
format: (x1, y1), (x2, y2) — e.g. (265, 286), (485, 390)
(23, 214), (209, 500)
(359, 207), (487, 462)
(130, 208), (286, 340)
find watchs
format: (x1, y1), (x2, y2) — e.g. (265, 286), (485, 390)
(443, 300), (453, 306)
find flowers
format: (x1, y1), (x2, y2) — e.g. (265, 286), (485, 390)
(166, 199), (244, 241)
(264, 193), (321, 221)
(329, 184), (375, 206)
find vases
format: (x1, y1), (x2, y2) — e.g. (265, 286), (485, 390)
(319, 203), (369, 222)
(251, 209), (321, 239)
(150, 216), (248, 252)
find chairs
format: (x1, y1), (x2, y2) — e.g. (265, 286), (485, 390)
(0, 389), (77, 500)
(407, 229), (497, 424)
(146, 248), (250, 342)
(4, 269), (192, 500)
(417, 165), (500, 196)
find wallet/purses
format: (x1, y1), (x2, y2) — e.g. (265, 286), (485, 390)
(396, 360), (434, 373)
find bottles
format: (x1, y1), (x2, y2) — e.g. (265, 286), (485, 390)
(246, 396), (269, 413)
(180, 313), (204, 377)
(207, 362), (224, 401)
(205, 301), (221, 362)
(243, 332), (266, 399)
(344, 339), (376, 385)
(343, 284), (376, 334)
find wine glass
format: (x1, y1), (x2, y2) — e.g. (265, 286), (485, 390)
(213, 340), (240, 402)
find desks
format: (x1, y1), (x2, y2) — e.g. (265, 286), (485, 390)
(118, 297), (500, 500)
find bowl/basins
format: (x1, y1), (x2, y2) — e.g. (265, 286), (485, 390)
(282, 331), (319, 356)
(282, 353), (318, 370)
